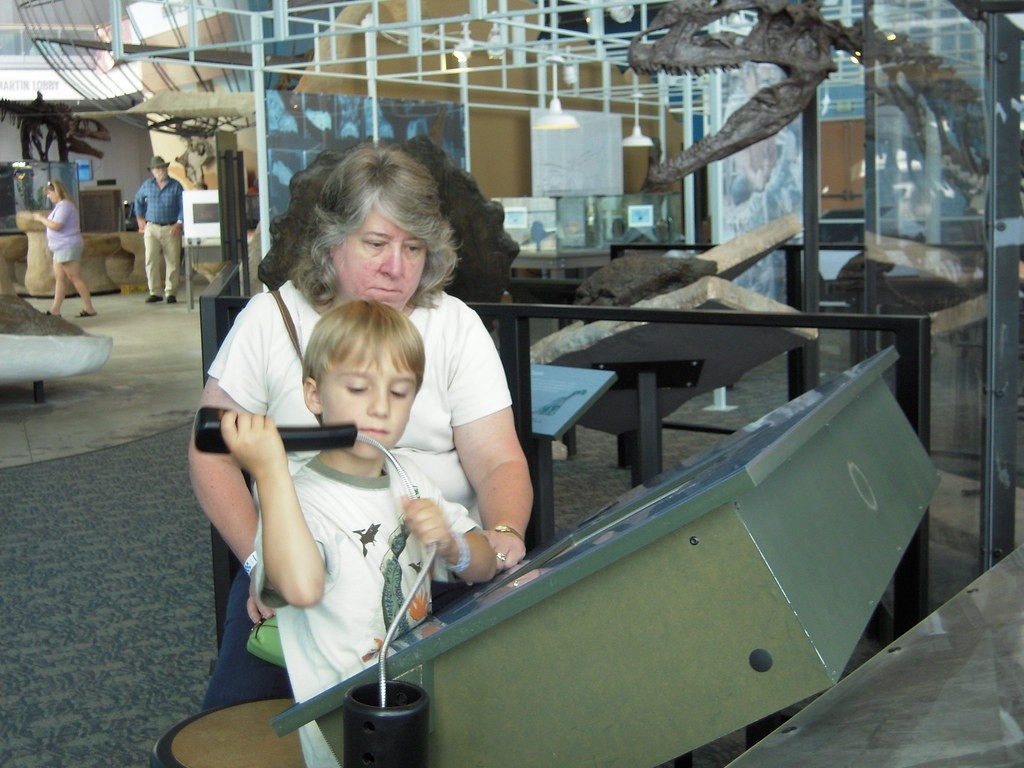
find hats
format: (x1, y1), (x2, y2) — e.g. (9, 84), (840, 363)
(146, 156), (171, 171)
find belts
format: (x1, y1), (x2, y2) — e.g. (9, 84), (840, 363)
(148, 221), (176, 226)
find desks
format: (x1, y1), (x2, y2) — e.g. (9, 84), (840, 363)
(513, 253), (641, 279)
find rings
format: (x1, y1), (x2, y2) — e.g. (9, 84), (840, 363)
(494, 551), (506, 561)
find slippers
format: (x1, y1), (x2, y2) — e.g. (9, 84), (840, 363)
(42, 310), (63, 317)
(75, 310), (98, 317)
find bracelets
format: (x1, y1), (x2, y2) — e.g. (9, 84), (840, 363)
(244, 552), (257, 576)
(445, 529), (471, 573)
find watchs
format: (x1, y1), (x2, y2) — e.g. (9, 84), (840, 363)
(493, 526), (524, 541)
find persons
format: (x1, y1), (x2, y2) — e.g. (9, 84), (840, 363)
(135, 157), (184, 304)
(189, 146), (535, 714)
(217, 299), (496, 768)
(34, 180), (97, 318)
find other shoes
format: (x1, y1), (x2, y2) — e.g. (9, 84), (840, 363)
(145, 295), (162, 303)
(167, 295), (177, 305)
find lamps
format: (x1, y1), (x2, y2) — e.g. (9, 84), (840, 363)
(486, 10), (506, 59)
(454, 15), (476, 63)
(610, 5), (635, 23)
(529, 62), (581, 130)
(620, 100), (655, 147)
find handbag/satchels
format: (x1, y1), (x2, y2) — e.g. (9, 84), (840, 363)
(248, 616), (288, 670)
